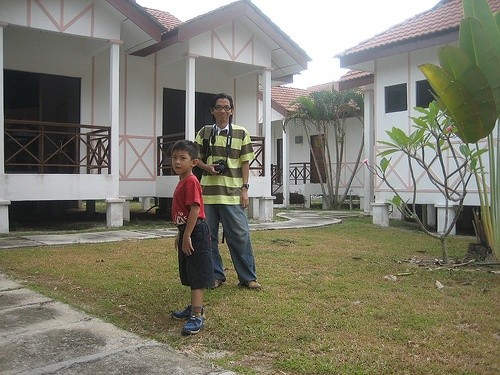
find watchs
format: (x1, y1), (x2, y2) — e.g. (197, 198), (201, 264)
(242, 183), (250, 189)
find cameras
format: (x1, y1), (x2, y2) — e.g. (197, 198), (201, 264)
(214, 159), (226, 171)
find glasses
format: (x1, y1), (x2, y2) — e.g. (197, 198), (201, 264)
(215, 105), (231, 111)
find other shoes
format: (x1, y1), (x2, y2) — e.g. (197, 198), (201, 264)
(241, 280), (261, 289)
(208, 280), (223, 288)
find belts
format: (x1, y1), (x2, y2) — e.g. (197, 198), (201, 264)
(177, 219), (205, 231)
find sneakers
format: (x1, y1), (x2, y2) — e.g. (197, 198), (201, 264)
(182, 307), (204, 334)
(171, 305), (191, 320)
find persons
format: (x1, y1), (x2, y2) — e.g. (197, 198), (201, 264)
(172, 140), (216, 335)
(193, 93), (262, 289)
